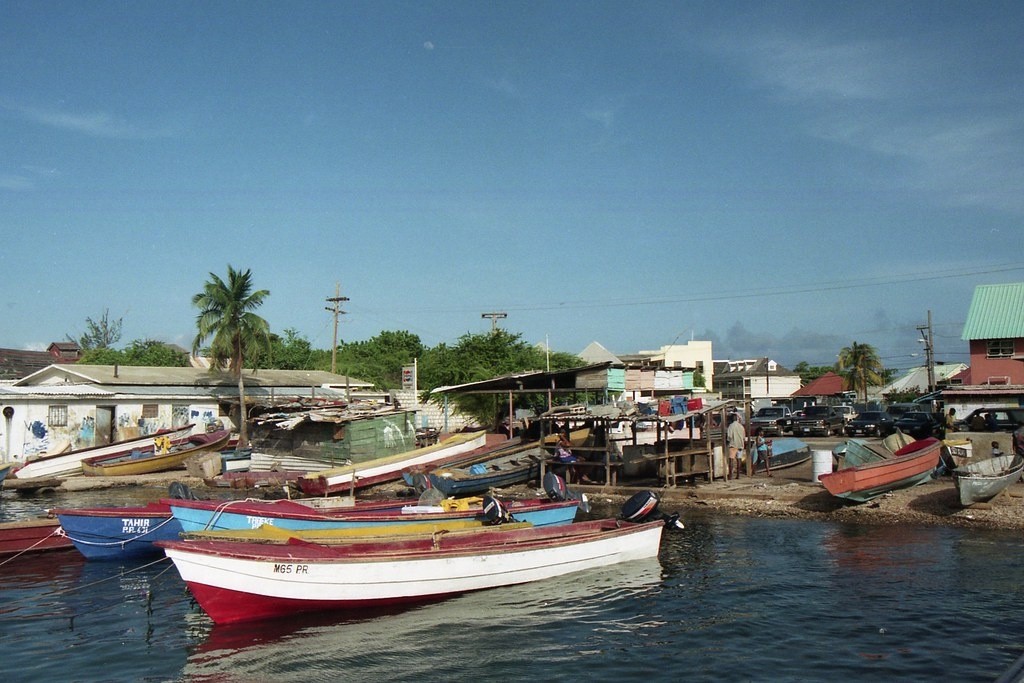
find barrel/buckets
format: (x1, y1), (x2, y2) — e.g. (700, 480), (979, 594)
(811, 450), (833, 483)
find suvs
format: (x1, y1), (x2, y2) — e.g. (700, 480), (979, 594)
(952, 408), (1024, 433)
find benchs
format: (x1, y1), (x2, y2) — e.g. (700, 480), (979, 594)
(629, 450), (713, 486)
(543, 445), (624, 486)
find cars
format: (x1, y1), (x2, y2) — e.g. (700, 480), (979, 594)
(794, 406), (848, 437)
(893, 411), (948, 440)
(844, 411), (895, 437)
(750, 406), (793, 437)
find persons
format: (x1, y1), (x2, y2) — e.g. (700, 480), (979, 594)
(971, 411), (998, 432)
(991, 441), (1004, 458)
(944, 408), (958, 434)
(726, 414), (745, 479)
(752, 427), (773, 477)
(552, 430), (593, 483)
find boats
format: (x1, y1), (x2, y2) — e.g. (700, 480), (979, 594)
(0, 422), (668, 624)
(744, 438), (812, 474)
(952, 455), (1024, 505)
(818, 437), (943, 502)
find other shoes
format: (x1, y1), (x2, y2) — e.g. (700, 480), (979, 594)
(769, 475), (773, 477)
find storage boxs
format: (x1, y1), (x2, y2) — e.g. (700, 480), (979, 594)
(401, 506), (431, 514)
(417, 506), (445, 513)
(183, 451), (222, 478)
(154, 436), (170, 456)
(470, 464), (487, 475)
